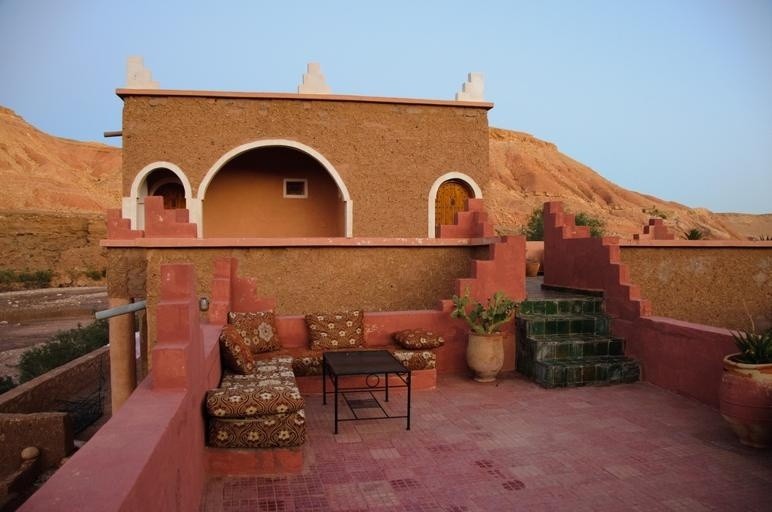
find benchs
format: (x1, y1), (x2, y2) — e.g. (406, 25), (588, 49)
(203, 342), (437, 475)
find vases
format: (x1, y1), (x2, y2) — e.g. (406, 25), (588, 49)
(526, 263), (542, 277)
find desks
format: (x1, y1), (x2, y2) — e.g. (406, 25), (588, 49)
(321, 350), (412, 435)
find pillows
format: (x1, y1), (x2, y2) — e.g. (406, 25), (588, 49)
(389, 327), (445, 350)
(204, 386), (306, 419)
(227, 309), (282, 354)
(305, 307), (368, 351)
(219, 324), (254, 376)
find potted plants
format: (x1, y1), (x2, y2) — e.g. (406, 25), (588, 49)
(712, 324), (772, 450)
(449, 288), (520, 383)
